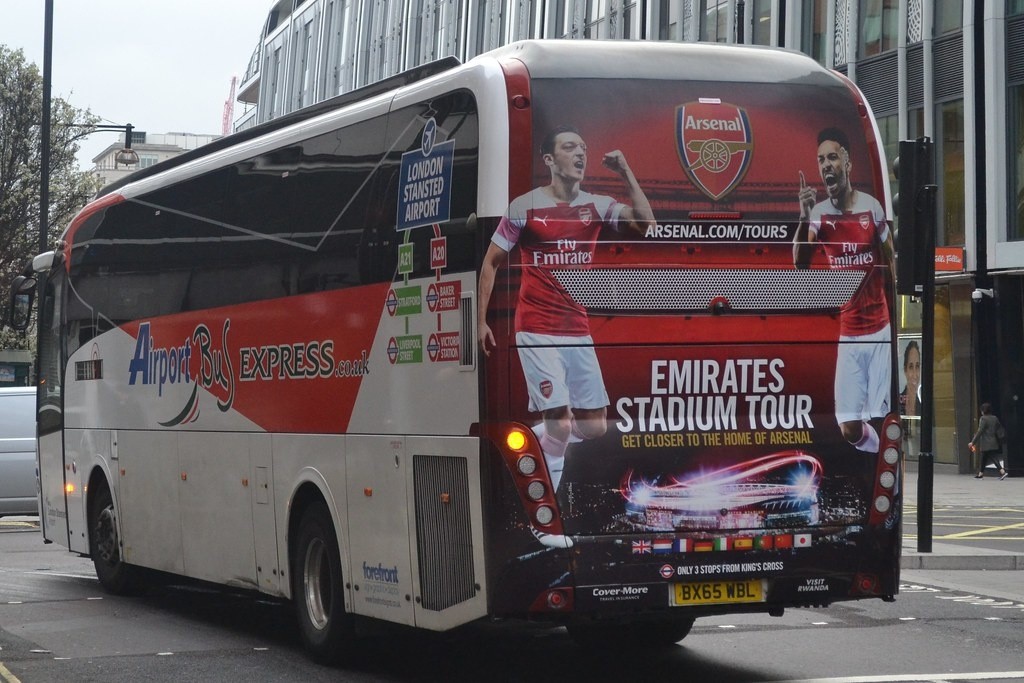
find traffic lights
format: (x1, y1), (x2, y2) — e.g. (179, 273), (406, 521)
(891, 137), (937, 298)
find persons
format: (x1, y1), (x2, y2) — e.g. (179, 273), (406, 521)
(793, 126), (892, 452)
(476, 125), (658, 550)
(900, 341), (921, 416)
(968, 403), (1009, 480)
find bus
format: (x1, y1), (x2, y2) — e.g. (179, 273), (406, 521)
(4, 40), (902, 668)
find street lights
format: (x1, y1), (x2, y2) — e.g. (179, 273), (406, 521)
(34, 0), (137, 345)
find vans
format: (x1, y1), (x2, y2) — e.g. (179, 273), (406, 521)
(0, 384), (38, 517)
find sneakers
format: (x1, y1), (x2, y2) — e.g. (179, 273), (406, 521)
(529, 524), (573, 548)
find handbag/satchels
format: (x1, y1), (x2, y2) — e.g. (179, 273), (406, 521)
(997, 425), (1007, 444)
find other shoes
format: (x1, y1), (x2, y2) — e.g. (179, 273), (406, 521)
(973, 475), (983, 480)
(1000, 472), (1008, 480)
